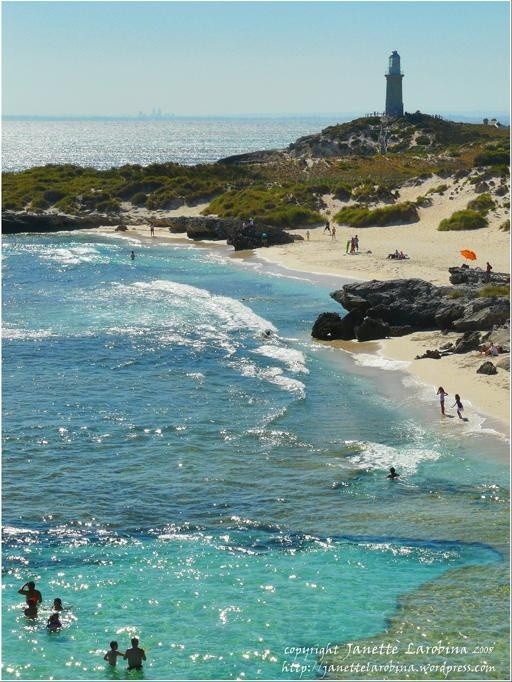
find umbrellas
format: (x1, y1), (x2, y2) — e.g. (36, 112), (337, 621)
(460, 249), (477, 260)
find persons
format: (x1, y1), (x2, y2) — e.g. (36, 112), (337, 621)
(349, 237), (356, 253)
(305, 230), (311, 240)
(486, 261), (492, 272)
(389, 249), (405, 261)
(332, 226), (337, 240)
(51, 598), (64, 611)
(480, 343), (501, 357)
(23, 600), (37, 618)
(123, 637), (147, 669)
(385, 467), (401, 479)
(323, 221), (331, 233)
(130, 251), (135, 260)
(104, 640), (125, 666)
(239, 216), (255, 229)
(354, 235), (359, 250)
(46, 615), (62, 628)
(18, 580), (43, 604)
(261, 231), (268, 246)
(150, 221), (154, 237)
(437, 386), (449, 416)
(451, 393), (464, 419)
(267, 229), (273, 246)
